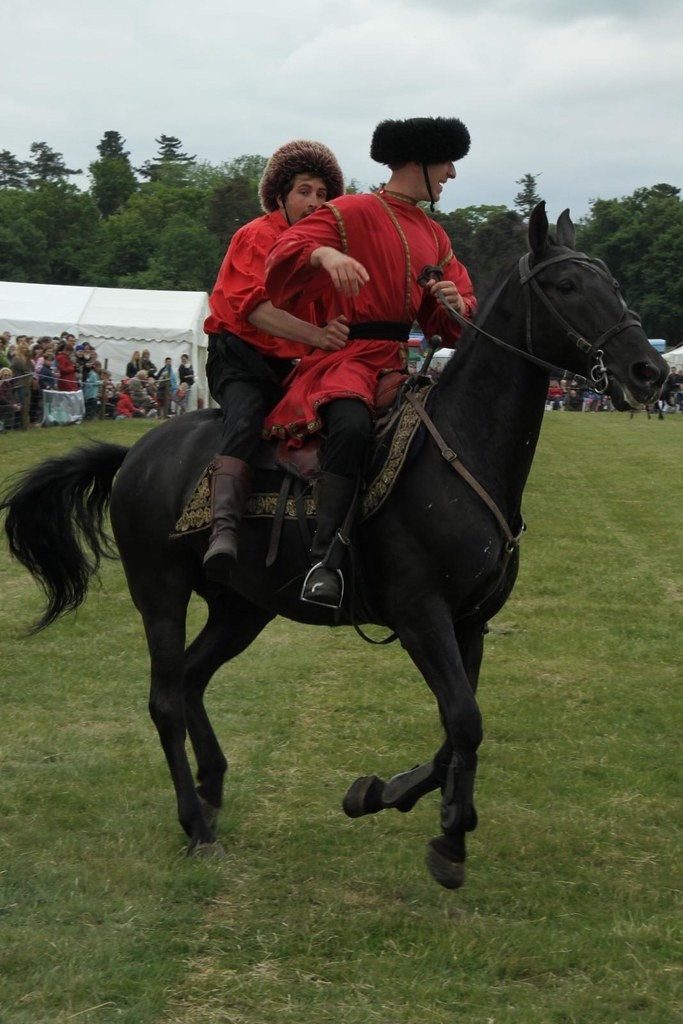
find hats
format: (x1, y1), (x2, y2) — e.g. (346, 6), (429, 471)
(76, 345), (85, 350)
(257, 140), (344, 216)
(370, 117), (473, 164)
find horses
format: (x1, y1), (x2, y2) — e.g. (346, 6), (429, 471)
(0, 199), (671, 890)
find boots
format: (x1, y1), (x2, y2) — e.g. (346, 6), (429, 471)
(203, 454), (254, 583)
(300, 470), (360, 604)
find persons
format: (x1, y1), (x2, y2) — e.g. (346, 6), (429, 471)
(545, 380), (604, 412)
(434, 361), (441, 377)
(266, 119), (477, 604)
(203, 140), (349, 563)
(0, 331), (195, 427)
(648, 367), (683, 414)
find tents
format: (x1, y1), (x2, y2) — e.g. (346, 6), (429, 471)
(662, 347), (683, 376)
(0, 280), (221, 411)
(407, 333), (456, 374)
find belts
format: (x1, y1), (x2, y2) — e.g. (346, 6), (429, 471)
(340, 322), (416, 342)
